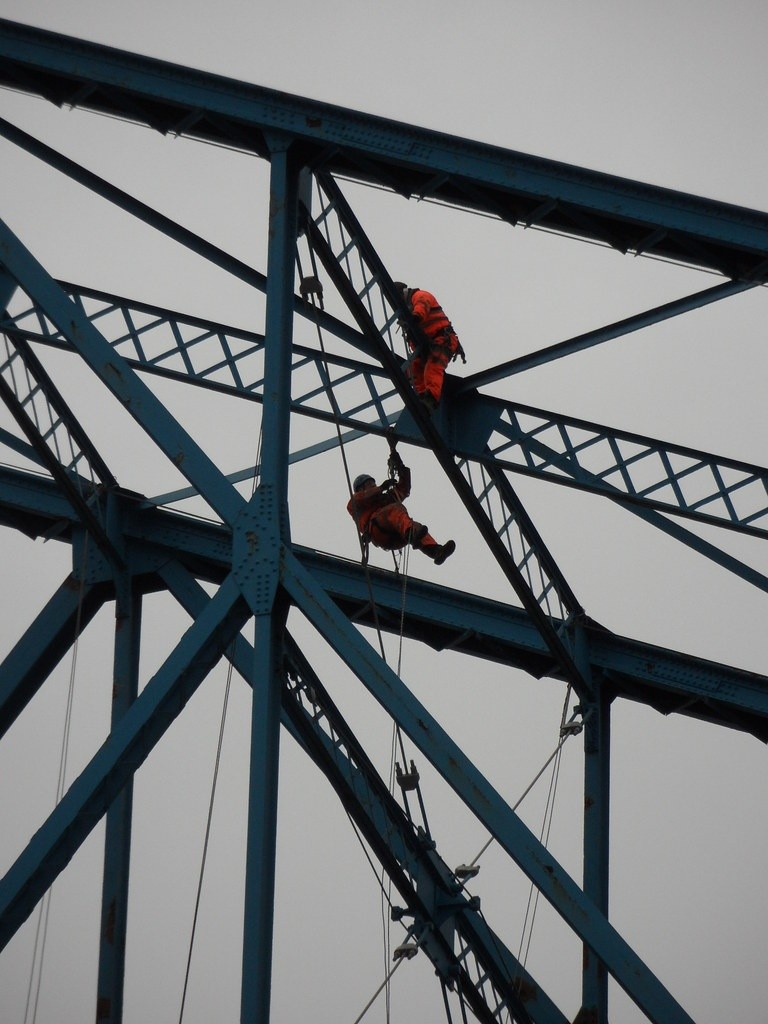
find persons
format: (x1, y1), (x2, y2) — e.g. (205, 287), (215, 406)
(347, 452), (456, 565)
(392, 281), (458, 417)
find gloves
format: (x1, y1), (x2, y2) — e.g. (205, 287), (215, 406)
(382, 477), (397, 490)
(390, 452), (404, 474)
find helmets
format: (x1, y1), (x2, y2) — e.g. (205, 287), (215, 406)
(394, 282), (407, 292)
(353, 474), (375, 493)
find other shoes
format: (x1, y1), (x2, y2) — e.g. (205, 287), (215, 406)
(413, 525), (428, 549)
(420, 392), (435, 411)
(434, 540), (456, 564)
